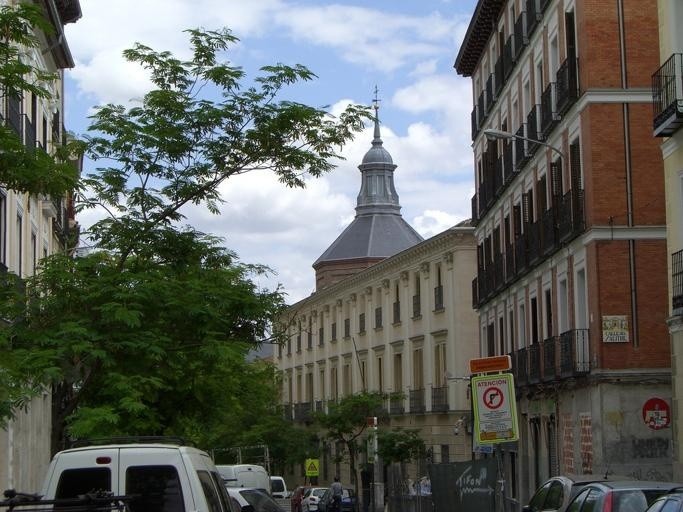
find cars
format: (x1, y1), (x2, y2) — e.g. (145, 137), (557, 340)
(300, 487), (327, 511)
(559, 479), (682, 511)
(317, 487), (358, 510)
(224, 486), (287, 511)
(520, 457), (646, 511)
(640, 486), (683, 512)
(291, 487), (310, 511)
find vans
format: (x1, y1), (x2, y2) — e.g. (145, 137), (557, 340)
(37, 435), (233, 510)
(215, 465), (271, 498)
(269, 476), (288, 500)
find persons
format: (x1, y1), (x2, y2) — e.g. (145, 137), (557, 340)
(330, 477), (344, 512)
(291, 484), (304, 512)
